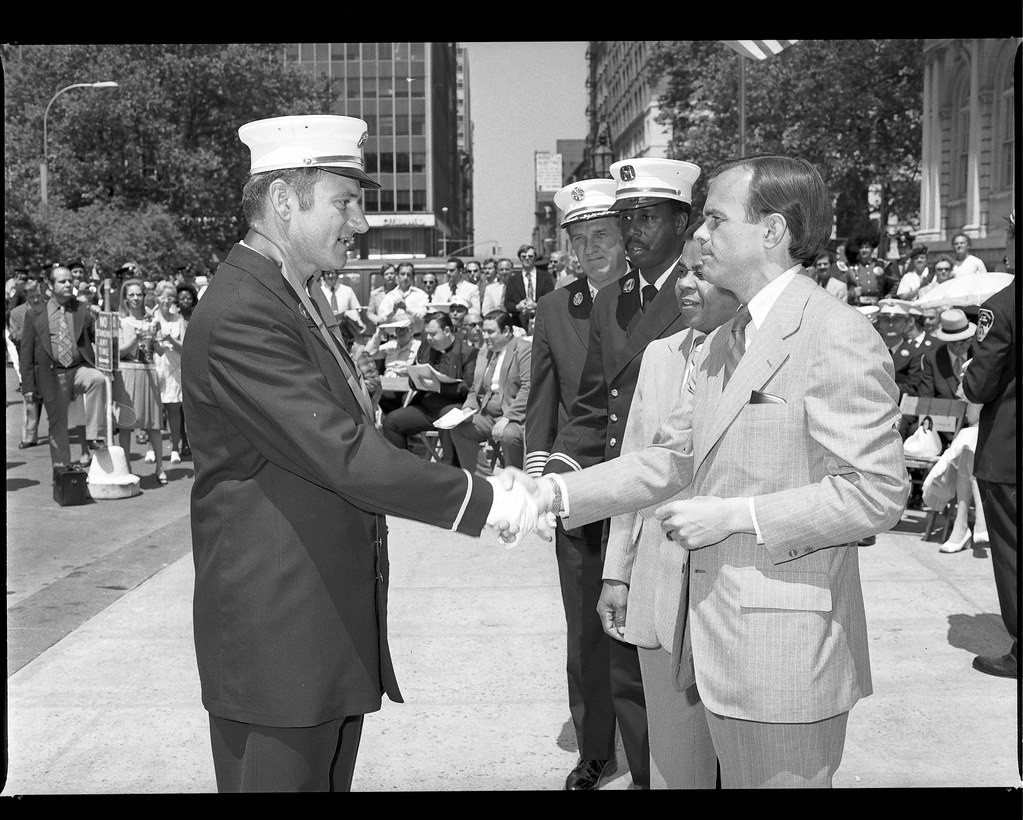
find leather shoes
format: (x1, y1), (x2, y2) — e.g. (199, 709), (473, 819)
(565, 758), (617, 791)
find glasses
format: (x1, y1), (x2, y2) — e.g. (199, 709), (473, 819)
(935, 267), (951, 273)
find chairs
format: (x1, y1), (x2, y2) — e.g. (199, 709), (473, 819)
(892, 391), (967, 546)
(369, 375), (506, 473)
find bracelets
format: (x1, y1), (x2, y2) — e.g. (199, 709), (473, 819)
(547, 476), (562, 516)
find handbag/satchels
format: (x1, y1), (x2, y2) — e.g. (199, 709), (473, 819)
(902, 415), (942, 457)
(52, 465), (88, 506)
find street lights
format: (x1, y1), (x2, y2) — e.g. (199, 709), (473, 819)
(40, 81), (118, 205)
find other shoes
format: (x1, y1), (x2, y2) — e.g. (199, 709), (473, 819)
(13, 382), (192, 486)
(858, 484), (1018, 681)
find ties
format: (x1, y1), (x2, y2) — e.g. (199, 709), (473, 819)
(956, 356), (965, 375)
(477, 351), (500, 415)
(450, 284), (457, 296)
(722, 304), (751, 392)
(58, 305), (73, 367)
(888, 348), (894, 355)
(428, 295), (432, 303)
(525, 273), (534, 300)
(910, 340), (918, 349)
(330, 287), (338, 312)
(639, 285), (658, 314)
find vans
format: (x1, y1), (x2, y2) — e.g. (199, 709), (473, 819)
(331, 258), (533, 344)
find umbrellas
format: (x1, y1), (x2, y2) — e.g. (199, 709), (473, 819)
(911, 271), (1016, 310)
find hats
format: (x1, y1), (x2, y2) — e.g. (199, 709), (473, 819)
(448, 295), (473, 314)
(936, 309), (978, 343)
(877, 297), (914, 318)
(607, 158), (702, 212)
(554, 178), (621, 229)
(237, 114), (383, 191)
(114, 266), (136, 280)
(907, 242), (928, 258)
(1002, 208), (1015, 226)
(174, 283), (199, 308)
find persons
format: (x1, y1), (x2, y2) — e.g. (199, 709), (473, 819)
(962, 210), (1018, 678)
(534, 158), (702, 790)
(804, 234), (990, 552)
(180, 115), (539, 793)
(597, 219), (739, 789)
(320, 245), (583, 476)
(4, 262), (209, 485)
(523, 179), (634, 791)
(485, 151), (912, 789)
